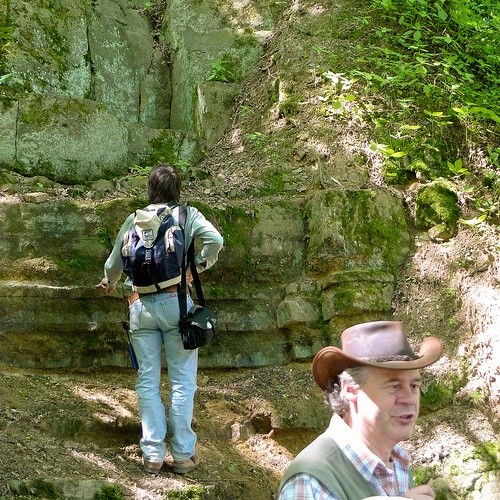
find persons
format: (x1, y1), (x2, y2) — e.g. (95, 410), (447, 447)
(94, 166), (224, 474)
(278, 319), (444, 500)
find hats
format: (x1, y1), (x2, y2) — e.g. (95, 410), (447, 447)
(312, 321), (442, 393)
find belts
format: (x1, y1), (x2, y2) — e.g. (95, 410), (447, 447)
(125, 285), (190, 304)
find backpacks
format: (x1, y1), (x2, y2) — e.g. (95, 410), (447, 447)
(120, 203), (185, 293)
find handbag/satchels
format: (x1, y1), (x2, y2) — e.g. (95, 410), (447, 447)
(180, 305), (218, 349)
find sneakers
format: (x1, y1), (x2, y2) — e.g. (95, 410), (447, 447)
(174, 453), (200, 473)
(140, 457), (164, 473)
